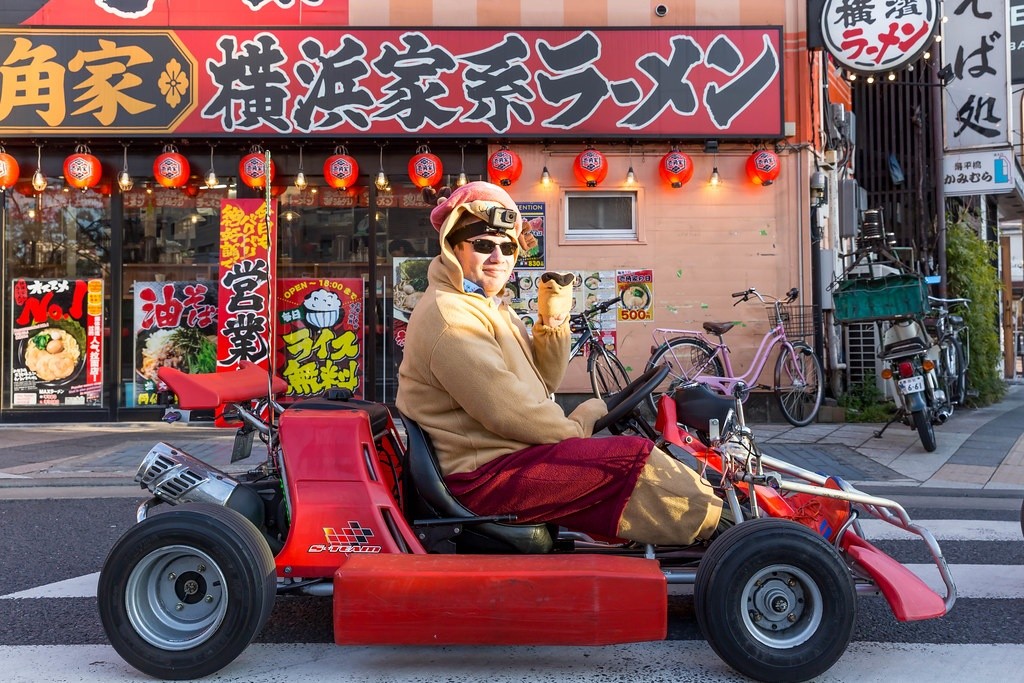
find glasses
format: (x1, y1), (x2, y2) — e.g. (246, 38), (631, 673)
(463, 238), (518, 256)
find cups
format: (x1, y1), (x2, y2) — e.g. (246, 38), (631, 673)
(155, 274), (165, 282)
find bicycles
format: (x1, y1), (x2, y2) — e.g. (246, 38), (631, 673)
(641, 286), (823, 431)
(566, 295), (641, 436)
(869, 268), (972, 454)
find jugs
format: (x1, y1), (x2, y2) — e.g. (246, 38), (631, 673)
(140, 235), (157, 263)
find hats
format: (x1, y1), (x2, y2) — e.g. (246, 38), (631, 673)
(430, 182), (522, 239)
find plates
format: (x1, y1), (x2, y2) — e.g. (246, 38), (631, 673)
(521, 316), (533, 328)
(528, 298), (538, 309)
(572, 275), (582, 287)
(519, 276), (532, 289)
(535, 277), (541, 288)
(585, 276), (601, 290)
(618, 283), (652, 313)
(393, 285), (412, 313)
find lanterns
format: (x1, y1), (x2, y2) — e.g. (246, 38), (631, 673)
(746, 148), (779, 186)
(153, 150), (190, 189)
(239, 150), (275, 191)
(488, 146), (522, 186)
(63, 152), (102, 191)
(574, 148), (608, 187)
(659, 149), (693, 188)
(324, 154), (359, 191)
(0, 151), (19, 190)
(407, 150), (443, 189)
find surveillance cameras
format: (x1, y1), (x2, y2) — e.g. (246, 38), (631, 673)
(938, 63), (952, 80)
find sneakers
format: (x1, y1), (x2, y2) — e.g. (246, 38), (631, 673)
(790, 476), (852, 544)
(779, 472), (828, 513)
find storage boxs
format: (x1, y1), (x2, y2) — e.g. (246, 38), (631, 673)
(833, 408), (847, 422)
(833, 273), (932, 323)
(818, 406), (832, 423)
(802, 403), (818, 423)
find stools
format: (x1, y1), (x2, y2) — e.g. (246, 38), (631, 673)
(365, 323), (389, 335)
(105, 326), (130, 338)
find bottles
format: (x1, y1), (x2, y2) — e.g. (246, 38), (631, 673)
(351, 246), (369, 263)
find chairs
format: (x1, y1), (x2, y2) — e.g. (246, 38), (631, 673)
(397, 408), (560, 555)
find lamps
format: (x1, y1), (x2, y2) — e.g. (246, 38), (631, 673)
(850, 62), (955, 87)
(365, 207), (387, 221)
(278, 194), (301, 221)
(703, 141), (719, 153)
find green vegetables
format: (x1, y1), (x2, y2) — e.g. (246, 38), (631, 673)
(187, 340), (217, 375)
(630, 288), (643, 298)
(32, 333), (52, 351)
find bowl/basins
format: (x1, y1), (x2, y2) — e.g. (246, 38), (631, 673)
(17, 319), (87, 387)
(137, 322), (205, 383)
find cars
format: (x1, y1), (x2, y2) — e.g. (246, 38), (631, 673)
(96, 359), (959, 682)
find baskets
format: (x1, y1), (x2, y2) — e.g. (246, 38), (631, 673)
(766, 305), (819, 337)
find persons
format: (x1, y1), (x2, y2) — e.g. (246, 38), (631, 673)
(387, 240), (415, 326)
(396, 181), (722, 545)
